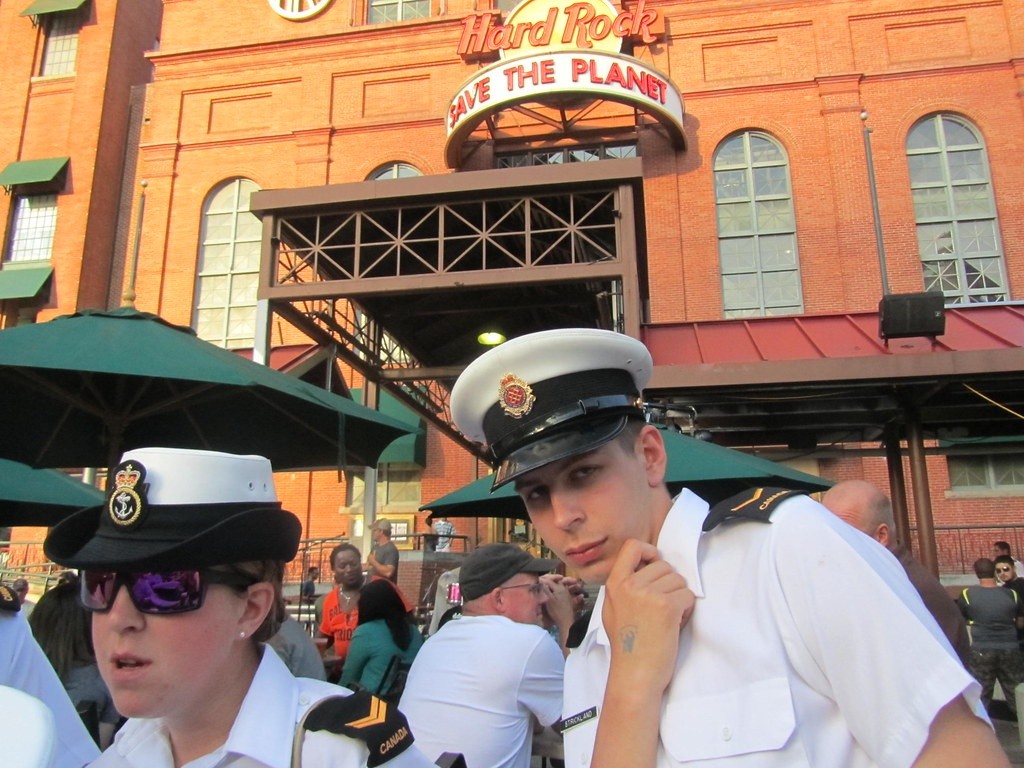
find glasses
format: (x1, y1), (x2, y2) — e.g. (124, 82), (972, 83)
(78, 567), (269, 613)
(995, 566), (1013, 573)
(311, 571), (318, 575)
(500, 583), (546, 594)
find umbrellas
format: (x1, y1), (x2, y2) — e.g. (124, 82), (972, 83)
(420, 423), (836, 522)
(0, 459), (105, 528)
(0, 307), (423, 470)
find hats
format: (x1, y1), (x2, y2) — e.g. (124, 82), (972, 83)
(450, 328), (654, 496)
(42, 447), (303, 572)
(459, 542), (562, 601)
(368, 518), (392, 530)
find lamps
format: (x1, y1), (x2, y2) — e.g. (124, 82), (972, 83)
(477, 318), (507, 346)
(878, 290), (945, 349)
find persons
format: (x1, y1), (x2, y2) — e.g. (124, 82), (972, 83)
(48, 570), (77, 589)
(316, 545), (414, 659)
(43, 447), (437, 767)
(396, 541), (583, 768)
(0, 585), (104, 767)
(337, 579), (424, 705)
(822, 481), (1023, 720)
(449, 328), (1011, 768)
(29, 583), (122, 753)
(368, 518), (400, 585)
(11, 578), (35, 617)
(302, 565), (322, 605)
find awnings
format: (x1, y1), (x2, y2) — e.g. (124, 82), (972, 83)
(939, 435), (1024, 455)
(19, 0), (91, 26)
(0, 157), (70, 191)
(0, 268), (54, 298)
(347, 384), (426, 469)
